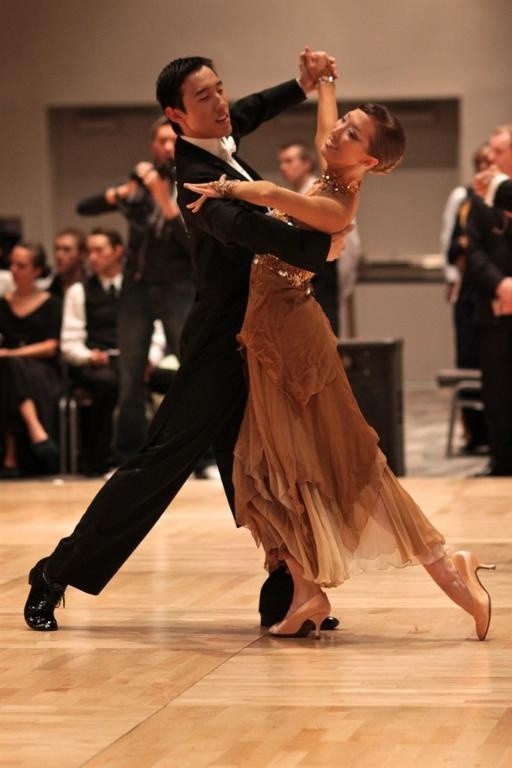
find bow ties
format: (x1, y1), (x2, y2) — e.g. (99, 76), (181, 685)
(219, 136), (238, 162)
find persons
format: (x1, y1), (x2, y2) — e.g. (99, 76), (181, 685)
(276, 140), (361, 308)
(76, 115), (211, 483)
(435, 137), (497, 457)
(461, 124), (512, 479)
(18, 45), (352, 635)
(176, 46), (499, 643)
(47, 231), (89, 297)
(59, 225), (181, 483)
(1, 237), (60, 480)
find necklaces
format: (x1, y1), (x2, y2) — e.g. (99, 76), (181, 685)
(312, 168), (361, 195)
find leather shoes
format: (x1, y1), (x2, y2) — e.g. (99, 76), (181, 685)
(24, 557), (65, 631)
(261, 615), (339, 629)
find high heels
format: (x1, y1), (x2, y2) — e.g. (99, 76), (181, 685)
(452, 551), (496, 641)
(269, 593), (331, 636)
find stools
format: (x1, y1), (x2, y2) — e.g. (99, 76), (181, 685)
(43, 379), (94, 476)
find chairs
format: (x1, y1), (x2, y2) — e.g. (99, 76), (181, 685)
(434, 367), (483, 459)
